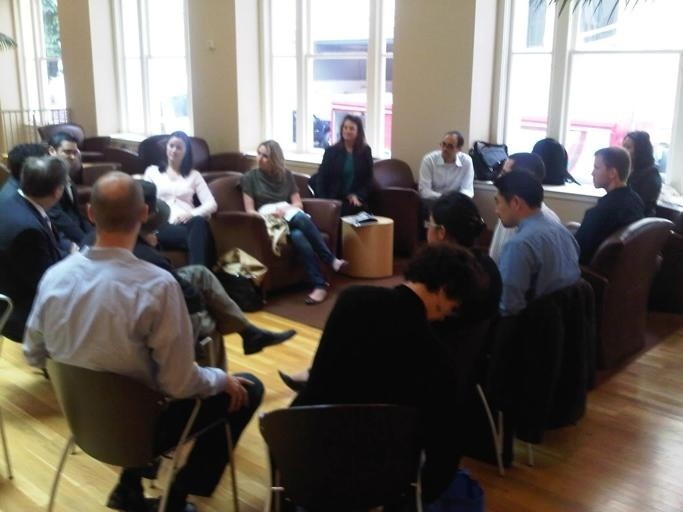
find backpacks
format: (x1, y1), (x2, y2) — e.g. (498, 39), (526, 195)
(531, 139), (581, 186)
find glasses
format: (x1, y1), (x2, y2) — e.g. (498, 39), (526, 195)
(424, 221), (442, 229)
(439, 142), (456, 151)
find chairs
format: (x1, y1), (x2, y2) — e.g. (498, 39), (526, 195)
(647, 205), (683, 315)
(0, 294), (15, 481)
(495, 262), (607, 469)
(372, 159), (419, 255)
(565, 218), (674, 373)
(260, 404), (431, 512)
(44, 337), (241, 511)
(444, 277), (516, 476)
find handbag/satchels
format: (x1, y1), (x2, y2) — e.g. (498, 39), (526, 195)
(470, 141), (509, 180)
(266, 215), (289, 258)
(216, 271), (264, 312)
(218, 247), (267, 286)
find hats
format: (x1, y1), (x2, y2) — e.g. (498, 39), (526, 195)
(137, 180), (170, 237)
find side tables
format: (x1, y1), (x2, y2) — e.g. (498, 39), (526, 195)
(342, 215), (393, 280)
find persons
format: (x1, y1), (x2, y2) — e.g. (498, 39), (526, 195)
(417, 130), (476, 200)
(80, 180), (297, 355)
(488, 130), (664, 318)
(143, 130), (220, 267)
(289, 243), (489, 511)
(316, 114), (373, 218)
(236, 140), (349, 304)
(426, 190), (502, 322)
(21, 170), (265, 512)
(0, 143), (79, 256)
(47, 132), (93, 240)
(0, 154), (70, 344)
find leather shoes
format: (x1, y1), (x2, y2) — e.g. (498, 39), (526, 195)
(107, 486), (159, 511)
(186, 502), (197, 512)
(304, 290), (328, 305)
(332, 259), (352, 272)
(279, 369), (309, 389)
(244, 329), (295, 354)
